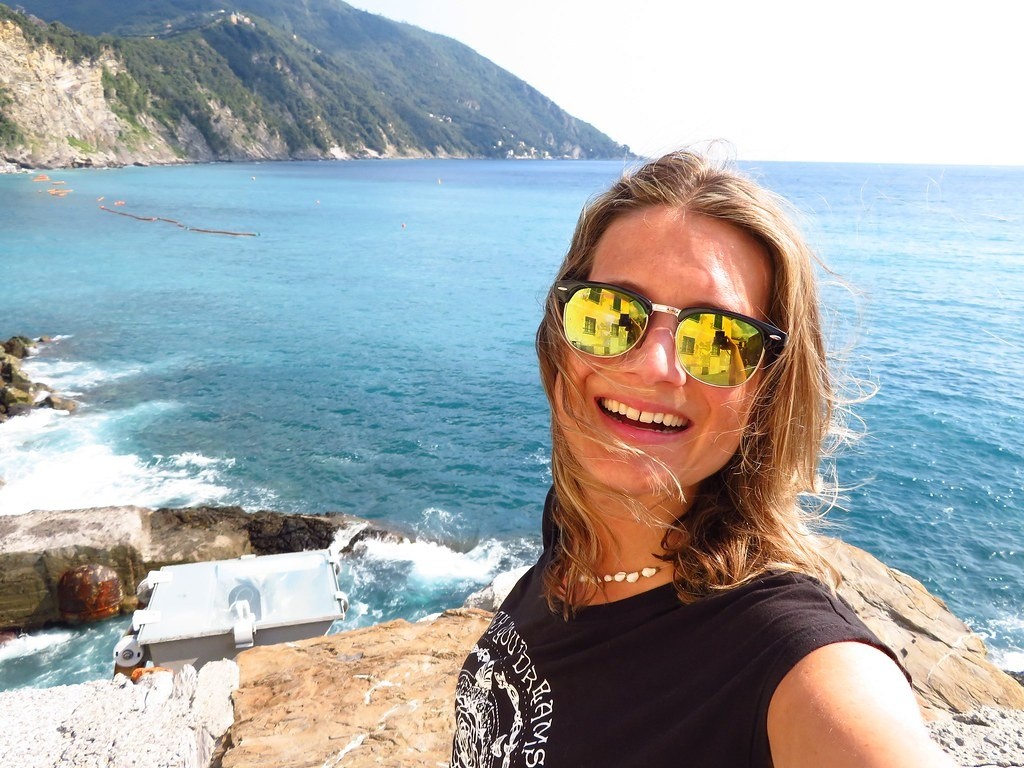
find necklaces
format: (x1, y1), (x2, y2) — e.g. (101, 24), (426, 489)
(576, 565), (671, 583)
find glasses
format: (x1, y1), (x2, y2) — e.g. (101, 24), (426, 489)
(553, 277), (786, 389)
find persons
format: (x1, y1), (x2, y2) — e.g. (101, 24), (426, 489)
(449, 149), (958, 768)
(713, 336), (747, 386)
(626, 317), (642, 340)
